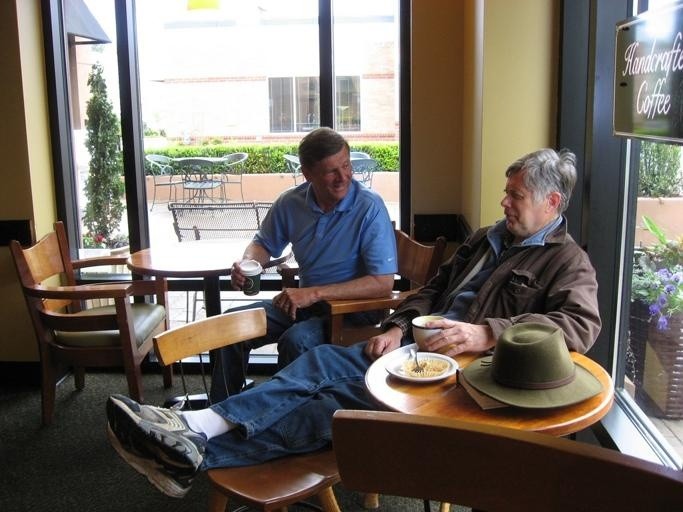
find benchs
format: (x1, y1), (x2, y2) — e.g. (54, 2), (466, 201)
(164, 200), (295, 275)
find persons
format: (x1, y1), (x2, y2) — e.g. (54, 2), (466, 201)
(106, 148), (602, 502)
(209, 128), (398, 408)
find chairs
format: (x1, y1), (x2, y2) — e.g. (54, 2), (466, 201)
(326, 404), (674, 511)
(6, 218), (174, 430)
(148, 308), (346, 512)
(274, 216), (448, 355)
(141, 143), (380, 218)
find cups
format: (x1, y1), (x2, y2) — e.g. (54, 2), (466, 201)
(239, 260), (263, 296)
(412, 314), (447, 353)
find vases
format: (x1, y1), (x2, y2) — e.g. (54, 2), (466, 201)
(80, 245), (133, 308)
(630, 299), (682, 421)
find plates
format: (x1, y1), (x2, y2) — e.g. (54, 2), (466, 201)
(385, 352), (459, 383)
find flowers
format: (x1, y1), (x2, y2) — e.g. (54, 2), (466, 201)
(81, 60), (124, 249)
(630, 261), (683, 332)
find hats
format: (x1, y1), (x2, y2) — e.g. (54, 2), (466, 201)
(462, 321), (605, 409)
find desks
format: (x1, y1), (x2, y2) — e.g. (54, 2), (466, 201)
(121, 238), (294, 412)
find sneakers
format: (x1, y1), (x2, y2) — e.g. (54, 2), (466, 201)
(106, 392), (209, 472)
(105, 421), (195, 499)
